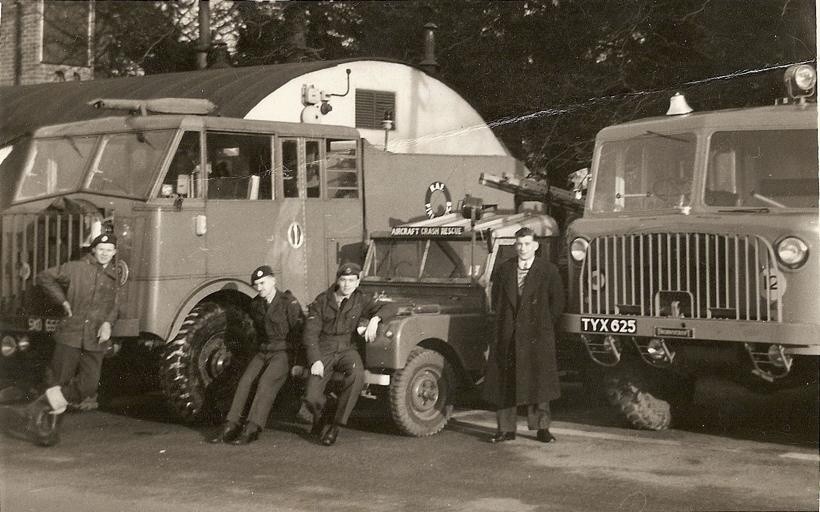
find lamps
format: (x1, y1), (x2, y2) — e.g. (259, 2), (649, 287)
(379, 109), (395, 151)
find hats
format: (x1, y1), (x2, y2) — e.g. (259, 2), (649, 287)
(335, 262), (363, 276)
(250, 266), (273, 287)
(90, 233), (119, 248)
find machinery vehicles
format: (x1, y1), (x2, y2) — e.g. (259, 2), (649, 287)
(554, 65), (820, 432)
(0, 94), (526, 426)
(289, 173), (616, 438)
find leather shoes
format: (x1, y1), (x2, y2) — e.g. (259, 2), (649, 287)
(488, 432), (517, 444)
(229, 426), (263, 446)
(537, 429), (556, 443)
(206, 423), (243, 444)
(308, 421), (324, 442)
(322, 426), (340, 447)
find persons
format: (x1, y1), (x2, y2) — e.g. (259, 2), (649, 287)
(302, 263), (399, 446)
(26, 233), (120, 446)
(210, 266), (307, 445)
(479, 227), (567, 443)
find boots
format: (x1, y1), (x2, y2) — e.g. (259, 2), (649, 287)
(20, 384), (69, 447)
